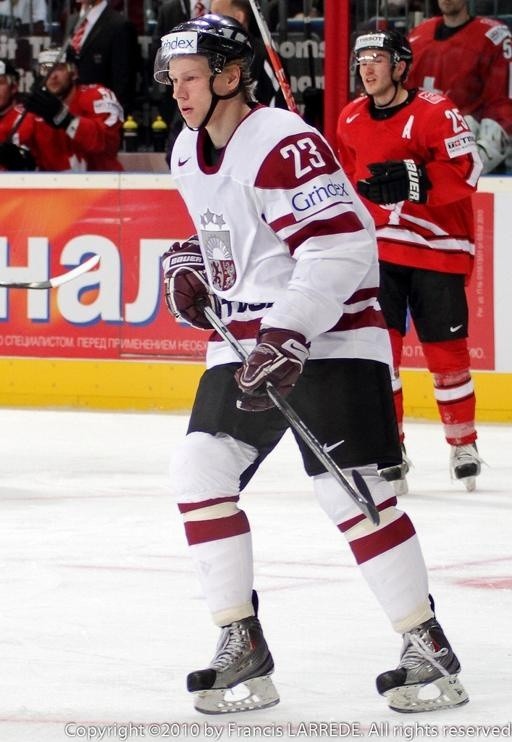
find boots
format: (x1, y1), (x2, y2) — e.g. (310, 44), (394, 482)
(186, 590), (276, 692)
(376, 595), (461, 694)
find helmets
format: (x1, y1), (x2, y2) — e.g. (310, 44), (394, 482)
(154, 15), (255, 87)
(351, 28), (413, 81)
(38, 42), (77, 66)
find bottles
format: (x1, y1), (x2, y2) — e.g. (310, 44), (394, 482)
(123, 116), (139, 153)
(152, 116), (168, 152)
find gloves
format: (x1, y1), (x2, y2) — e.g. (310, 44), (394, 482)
(0, 142), (35, 171)
(234, 328), (311, 412)
(161, 235), (223, 329)
(15, 89), (70, 128)
(356, 158), (432, 204)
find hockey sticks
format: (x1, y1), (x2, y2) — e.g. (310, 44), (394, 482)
(195, 296), (379, 526)
(6, 0), (95, 143)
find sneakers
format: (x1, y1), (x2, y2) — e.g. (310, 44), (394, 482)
(379, 442), (410, 482)
(452, 440), (480, 480)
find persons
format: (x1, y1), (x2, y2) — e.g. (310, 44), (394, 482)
(335, 23), (484, 485)
(151, 13), (462, 701)
(400, 2), (511, 176)
(0, 2), (288, 177)
